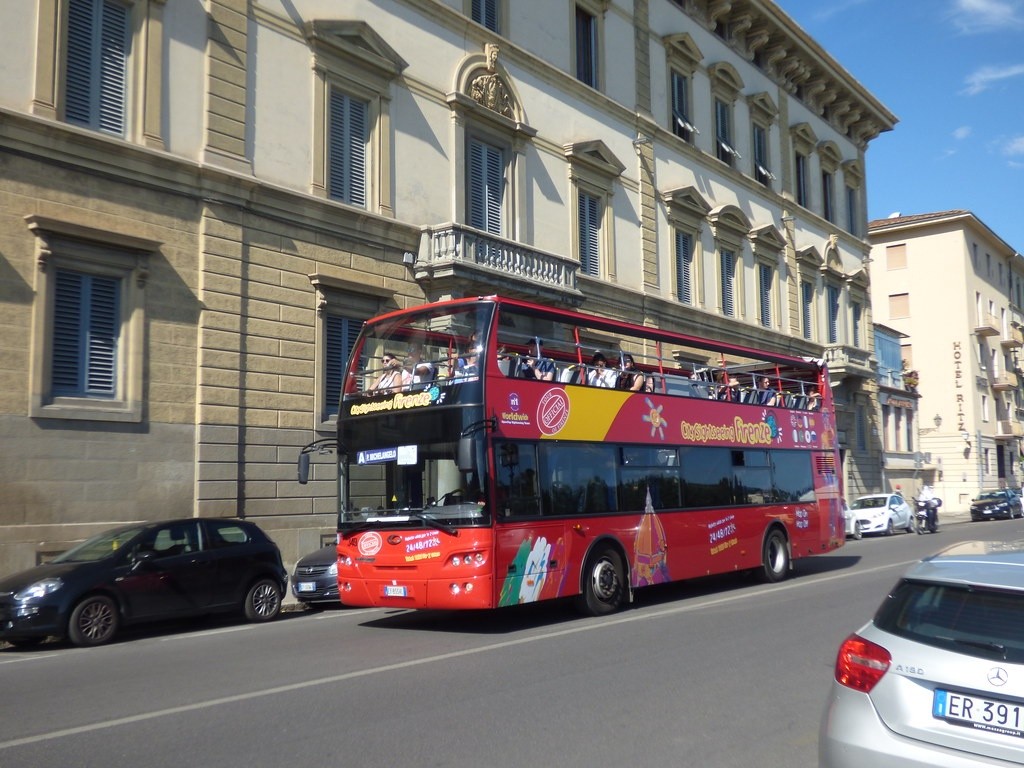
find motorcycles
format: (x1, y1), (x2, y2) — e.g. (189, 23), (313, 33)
(912, 496), (943, 535)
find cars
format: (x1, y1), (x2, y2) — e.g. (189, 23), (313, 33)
(850, 494), (915, 535)
(841, 497), (862, 539)
(970, 489), (1024, 521)
(0, 517), (288, 647)
(291, 538), (341, 611)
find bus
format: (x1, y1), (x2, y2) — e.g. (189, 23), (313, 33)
(299, 294), (846, 617)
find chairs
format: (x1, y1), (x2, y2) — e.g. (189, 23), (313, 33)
(396, 351), (816, 412)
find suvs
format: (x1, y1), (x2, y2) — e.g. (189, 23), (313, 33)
(818, 540), (1024, 768)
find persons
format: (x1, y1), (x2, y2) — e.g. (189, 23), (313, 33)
(499, 345), (517, 367)
(617, 354), (644, 391)
(587, 353), (616, 388)
(918, 486), (937, 521)
(744, 377), (776, 405)
(895, 485), (902, 496)
(688, 372), (706, 397)
(805, 385), (821, 410)
(715, 372), (741, 401)
(366, 329), (484, 397)
(522, 337), (555, 381)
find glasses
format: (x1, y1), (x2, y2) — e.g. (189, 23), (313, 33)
(381, 359), (389, 363)
(624, 358), (631, 363)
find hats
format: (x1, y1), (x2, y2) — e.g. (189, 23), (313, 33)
(526, 338), (544, 345)
